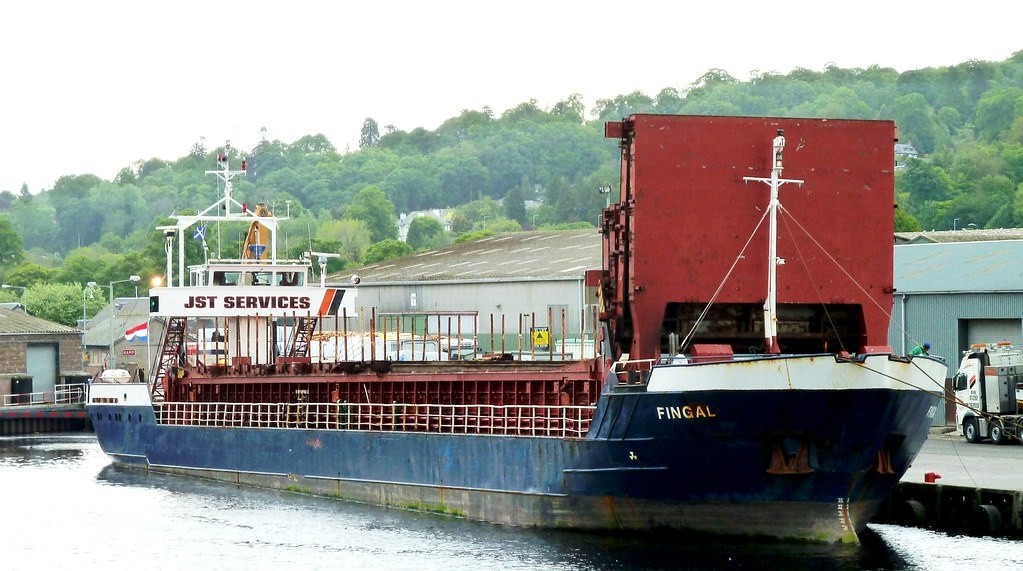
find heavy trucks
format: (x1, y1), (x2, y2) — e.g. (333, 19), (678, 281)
(951, 341), (1023, 444)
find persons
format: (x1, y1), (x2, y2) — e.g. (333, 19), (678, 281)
(907, 342), (931, 356)
(83, 352), (89, 366)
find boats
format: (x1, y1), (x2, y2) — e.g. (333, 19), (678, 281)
(86, 113), (947, 549)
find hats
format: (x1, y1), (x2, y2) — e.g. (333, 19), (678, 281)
(924, 342), (932, 349)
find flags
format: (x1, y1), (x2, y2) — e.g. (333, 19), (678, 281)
(124, 321), (146, 344)
(193, 226), (204, 240)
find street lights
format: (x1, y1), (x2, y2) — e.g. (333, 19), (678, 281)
(954, 218), (960, 230)
(597, 214), (602, 227)
(1, 284), (27, 314)
(484, 216), (489, 229)
(83, 287), (94, 351)
(87, 275), (142, 370)
(532, 215), (539, 227)
